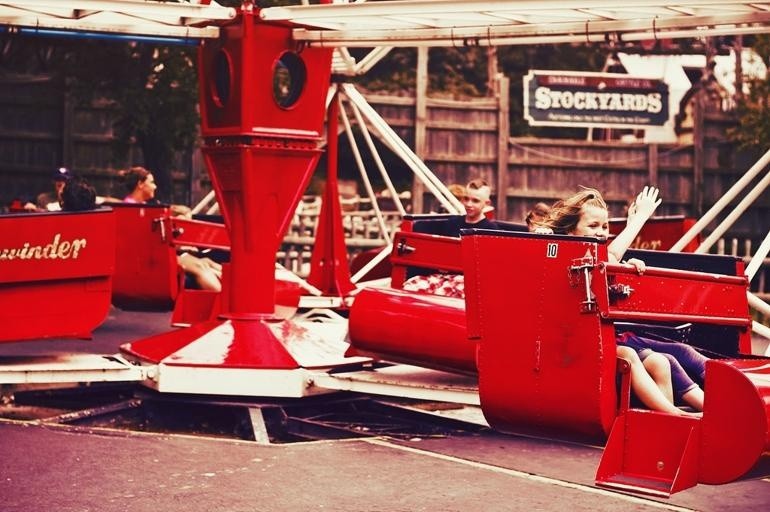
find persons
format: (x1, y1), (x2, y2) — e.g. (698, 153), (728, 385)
(10, 164), (222, 292)
(527, 185), (711, 418)
(442, 179), (500, 238)
